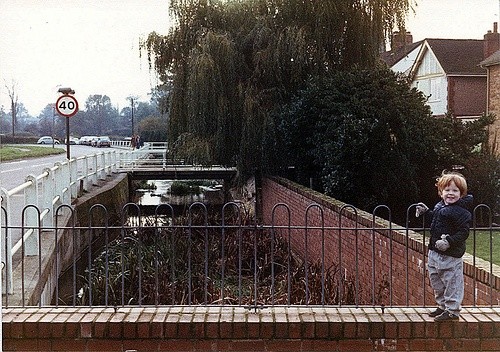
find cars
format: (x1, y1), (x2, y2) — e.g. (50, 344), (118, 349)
(64, 135), (111, 148)
(36, 135), (61, 145)
(121, 136), (132, 145)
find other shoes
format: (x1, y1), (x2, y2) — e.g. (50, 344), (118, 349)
(428, 307), (459, 322)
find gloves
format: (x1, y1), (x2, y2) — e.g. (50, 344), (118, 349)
(417, 203), (429, 215)
(435, 240), (450, 252)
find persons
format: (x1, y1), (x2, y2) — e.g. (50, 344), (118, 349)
(415, 172), (473, 323)
(131, 134), (144, 151)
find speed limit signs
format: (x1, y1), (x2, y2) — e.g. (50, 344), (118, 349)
(55, 95), (79, 117)
